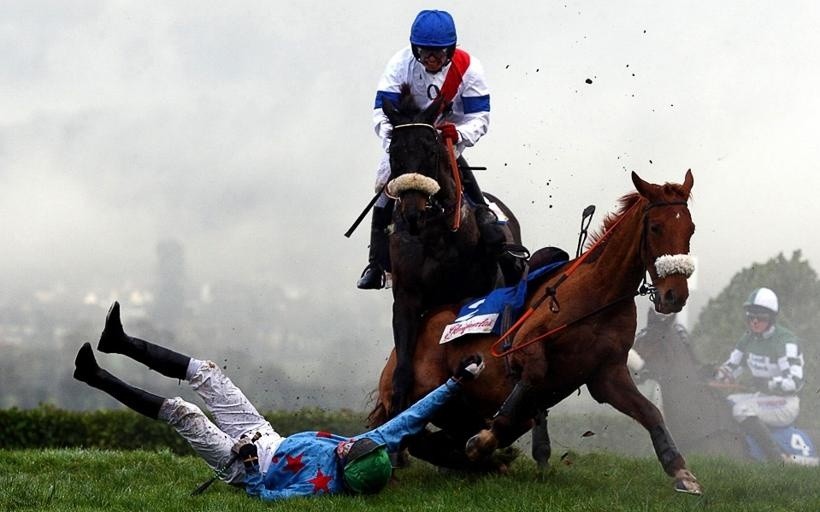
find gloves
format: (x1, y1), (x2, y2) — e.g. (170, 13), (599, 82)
(436, 124), (458, 144)
(740, 375), (769, 394)
(453, 351), (486, 387)
(232, 434), (258, 467)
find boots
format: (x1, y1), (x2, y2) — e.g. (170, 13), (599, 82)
(98, 301), (191, 380)
(74, 343), (166, 420)
(357, 206), (392, 289)
(452, 154), (496, 226)
(740, 415), (791, 463)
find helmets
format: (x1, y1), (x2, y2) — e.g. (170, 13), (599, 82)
(743, 287), (779, 316)
(410, 9), (458, 47)
(344, 438), (393, 495)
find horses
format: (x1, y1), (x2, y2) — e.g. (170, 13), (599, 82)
(377, 82), (551, 481)
(363, 169), (703, 494)
(627, 307), (790, 468)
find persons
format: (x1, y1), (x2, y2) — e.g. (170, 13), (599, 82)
(73, 300), (486, 501)
(717, 290), (804, 467)
(355, 9), (525, 290)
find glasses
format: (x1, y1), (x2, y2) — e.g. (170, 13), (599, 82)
(418, 48), (447, 58)
(745, 310), (771, 321)
